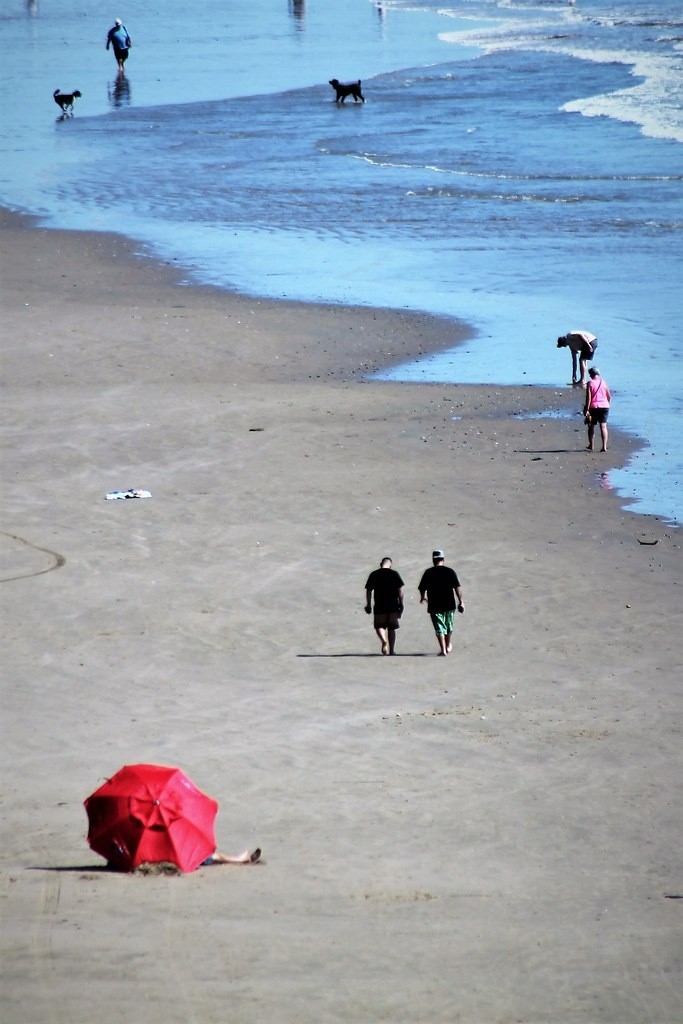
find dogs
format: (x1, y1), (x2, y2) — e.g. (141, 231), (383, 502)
(329, 79), (366, 103)
(53, 89), (82, 114)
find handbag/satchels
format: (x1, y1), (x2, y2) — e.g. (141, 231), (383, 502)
(583, 404), (588, 416)
(123, 26), (131, 47)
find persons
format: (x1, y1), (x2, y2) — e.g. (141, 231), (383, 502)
(200, 848), (261, 864)
(106, 18), (132, 71)
(364, 557), (404, 655)
(583, 366), (610, 452)
(557, 331), (598, 385)
(418, 549), (464, 657)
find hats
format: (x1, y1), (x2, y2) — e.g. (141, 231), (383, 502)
(557, 337), (565, 348)
(432, 549), (444, 559)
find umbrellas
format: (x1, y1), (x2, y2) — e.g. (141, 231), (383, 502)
(83, 764), (218, 874)
